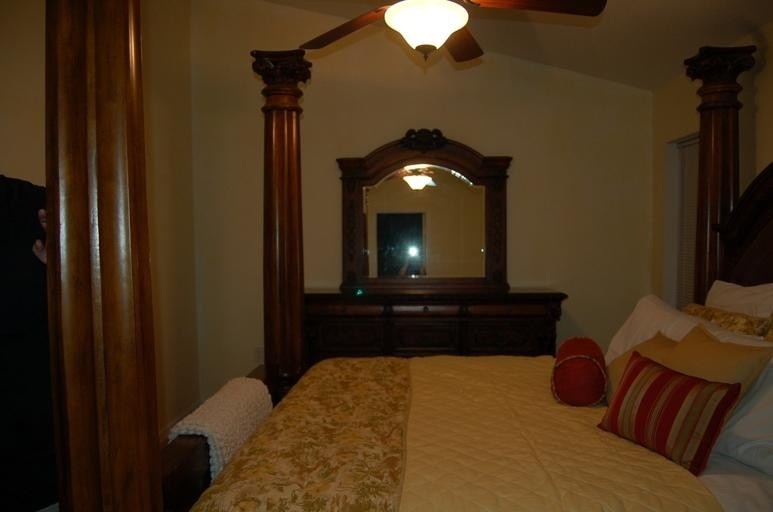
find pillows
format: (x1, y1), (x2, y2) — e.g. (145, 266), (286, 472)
(596, 350), (744, 477)
(548, 336), (608, 408)
(605, 278), (773, 473)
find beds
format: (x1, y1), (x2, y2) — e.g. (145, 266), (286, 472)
(162, 160), (773, 510)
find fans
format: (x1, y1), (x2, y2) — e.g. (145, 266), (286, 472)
(299, 0), (606, 61)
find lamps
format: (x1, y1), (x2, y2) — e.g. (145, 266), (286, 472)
(400, 167), (435, 189)
(384, 0), (470, 58)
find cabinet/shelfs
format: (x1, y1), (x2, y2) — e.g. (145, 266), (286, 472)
(295, 288), (567, 355)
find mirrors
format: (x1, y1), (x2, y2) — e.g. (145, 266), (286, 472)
(333, 126), (509, 295)
